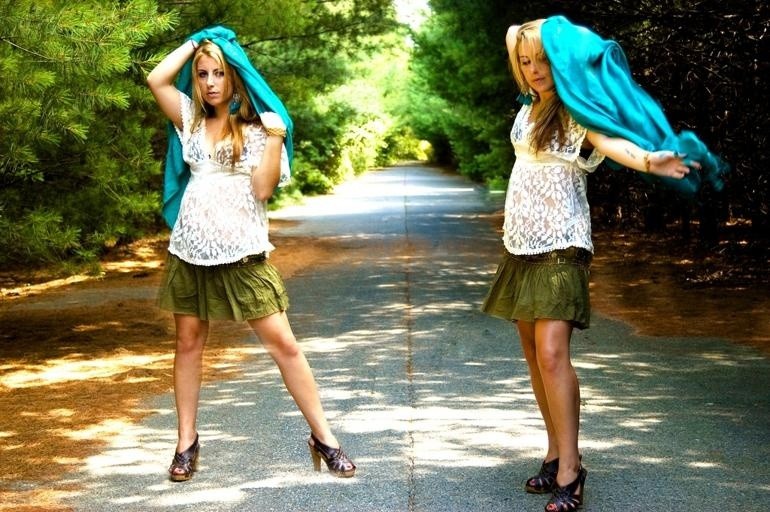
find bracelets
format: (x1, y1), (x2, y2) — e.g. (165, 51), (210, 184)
(266, 128), (288, 139)
(190, 39), (198, 51)
(642, 152), (652, 174)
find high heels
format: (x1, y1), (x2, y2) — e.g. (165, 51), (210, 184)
(169, 435), (200, 481)
(306, 433), (358, 477)
(525, 452), (587, 511)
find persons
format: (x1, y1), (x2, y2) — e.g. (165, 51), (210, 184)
(144, 22), (360, 484)
(476, 17), (704, 511)
